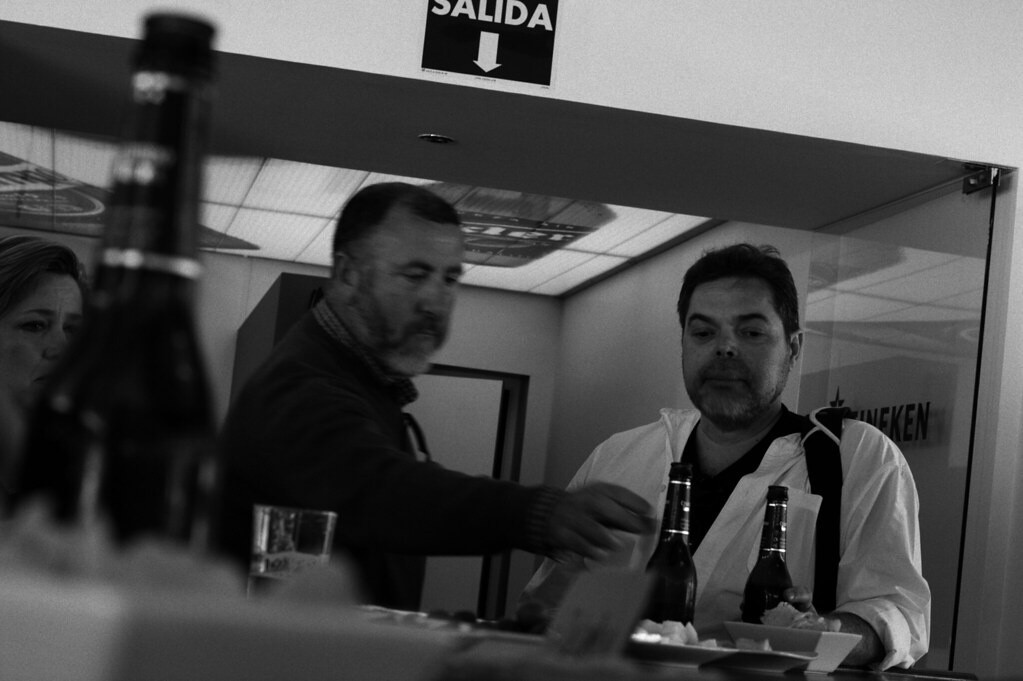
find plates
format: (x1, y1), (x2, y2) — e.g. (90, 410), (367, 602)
(370, 625), (491, 656)
(627, 639), (732, 670)
(729, 650), (820, 673)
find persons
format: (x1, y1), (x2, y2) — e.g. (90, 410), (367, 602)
(0, 235), (85, 522)
(206, 182), (655, 612)
(519, 242), (932, 673)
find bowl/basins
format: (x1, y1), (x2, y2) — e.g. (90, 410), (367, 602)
(721, 619), (865, 675)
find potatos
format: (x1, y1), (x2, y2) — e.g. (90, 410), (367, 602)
(629, 617), (772, 650)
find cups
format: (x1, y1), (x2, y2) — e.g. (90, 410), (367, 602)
(248, 504), (339, 606)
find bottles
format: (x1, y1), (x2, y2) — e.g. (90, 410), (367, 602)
(643, 459), (697, 625)
(739, 484), (798, 620)
